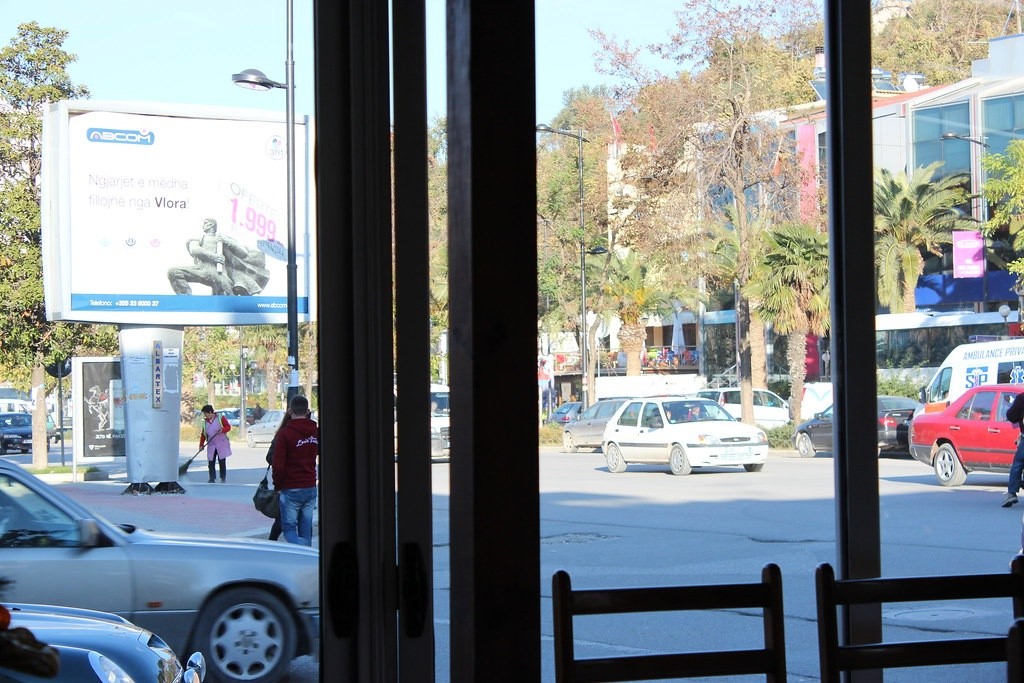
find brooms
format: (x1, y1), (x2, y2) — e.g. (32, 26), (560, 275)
(179, 429), (221, 477)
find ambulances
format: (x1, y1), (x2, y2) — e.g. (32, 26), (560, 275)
(913, 335), (1024, 420)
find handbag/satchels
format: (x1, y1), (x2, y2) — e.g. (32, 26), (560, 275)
(252, 477), (280, 518)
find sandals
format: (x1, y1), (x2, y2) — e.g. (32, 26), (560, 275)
(207, 479), (215, 483)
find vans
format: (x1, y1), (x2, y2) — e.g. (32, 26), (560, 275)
(787, 382), (833, 418)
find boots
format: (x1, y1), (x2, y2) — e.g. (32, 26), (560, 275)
(269, 522), (283, 541)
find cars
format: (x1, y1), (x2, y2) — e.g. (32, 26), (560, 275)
(601, 397), (769, 477)
(0, 460), (322, 682)
(907, 384), (1024, 485)
(214, 408), (255, 427)
(0, 412), (50, 455)
(45, 414), (60, 443)
(550, 401), (583, 425)
(562, 397), (659, 455)
(246, 410), (318, 448)
(393, 384), (450, 460)
(694, 388), (791, 429)
(0, 599), (207, 682)
(792, 396), (920, 458)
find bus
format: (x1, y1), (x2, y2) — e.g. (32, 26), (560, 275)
(876, 311), (1024, 383)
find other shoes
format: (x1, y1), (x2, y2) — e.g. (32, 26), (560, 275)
(220, 478), (226, 483)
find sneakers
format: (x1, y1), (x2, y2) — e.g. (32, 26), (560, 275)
(1002, 494), (1018, 507)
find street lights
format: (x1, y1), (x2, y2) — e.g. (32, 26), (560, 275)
(231, 67), (300, 398)
(998, 304), (1011, 337)
(537, 122), (608, 409)
(941, 130), (988, 312)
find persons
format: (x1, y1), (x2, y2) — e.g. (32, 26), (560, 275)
(199, 405), (232, 483)
(652, 403), (708, 431)
(1002, 392), (1024, 507)
(266, 395), (319, 547)
